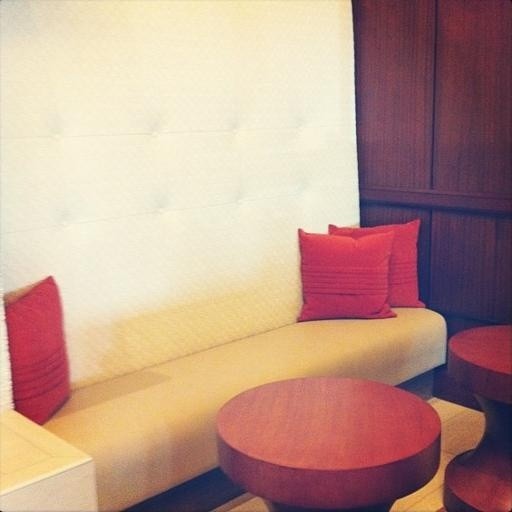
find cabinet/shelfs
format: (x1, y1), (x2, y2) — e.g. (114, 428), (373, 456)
(352, 1), (511, 324)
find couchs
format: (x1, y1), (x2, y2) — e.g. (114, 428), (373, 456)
(27, 278), (447, 512)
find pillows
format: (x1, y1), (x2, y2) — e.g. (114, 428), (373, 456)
(298, 229), (396, 322)
(4, 275), (73, 423)
(329, 218), (427, 308)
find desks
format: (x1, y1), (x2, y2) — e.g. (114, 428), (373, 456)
(443, 325), (511, 511)
(1, 411), (99, 511)
(216, 375), (442, 512)
